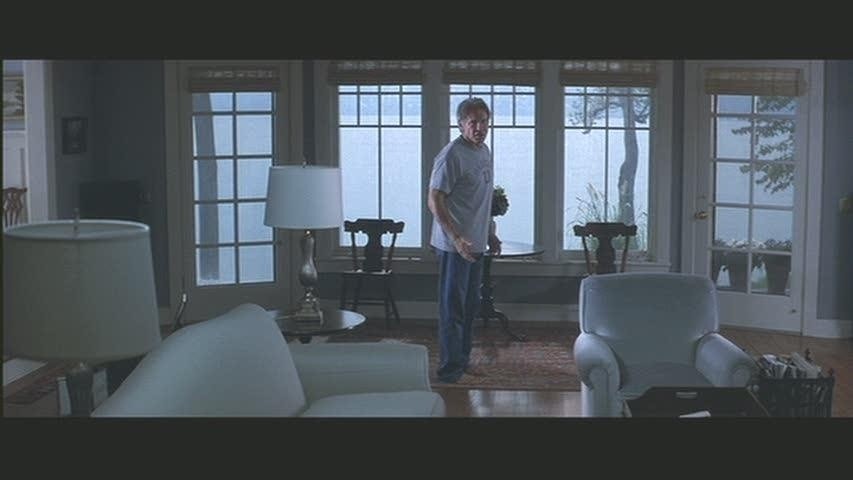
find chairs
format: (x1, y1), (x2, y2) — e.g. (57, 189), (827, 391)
(573, 222), (637, 274)
(573, 273), (757, 418)
(344, 219), (404, 329)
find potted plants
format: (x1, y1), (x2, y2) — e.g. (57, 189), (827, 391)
(486, 185), (509, 250)
(712, 237), (793, 294)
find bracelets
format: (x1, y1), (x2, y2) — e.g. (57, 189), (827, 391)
(452, 236), (462, 244)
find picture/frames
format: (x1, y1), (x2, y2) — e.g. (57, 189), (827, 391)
(62, 116), (88, 153)
(3, 76), (25, 120)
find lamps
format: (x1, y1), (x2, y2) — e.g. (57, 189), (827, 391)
(2, 207), (161, 419)
(264, 161), (342, 323)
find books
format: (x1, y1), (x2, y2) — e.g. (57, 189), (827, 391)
(757, 351), (823, 404)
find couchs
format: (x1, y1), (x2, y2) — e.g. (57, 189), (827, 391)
(87, 304), (445, 419)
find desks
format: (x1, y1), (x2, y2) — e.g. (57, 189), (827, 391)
(475, 241), (545, 341)
(267, 309), (367, 343)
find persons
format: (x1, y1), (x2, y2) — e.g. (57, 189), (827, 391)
(428, 97), (501, 383)
